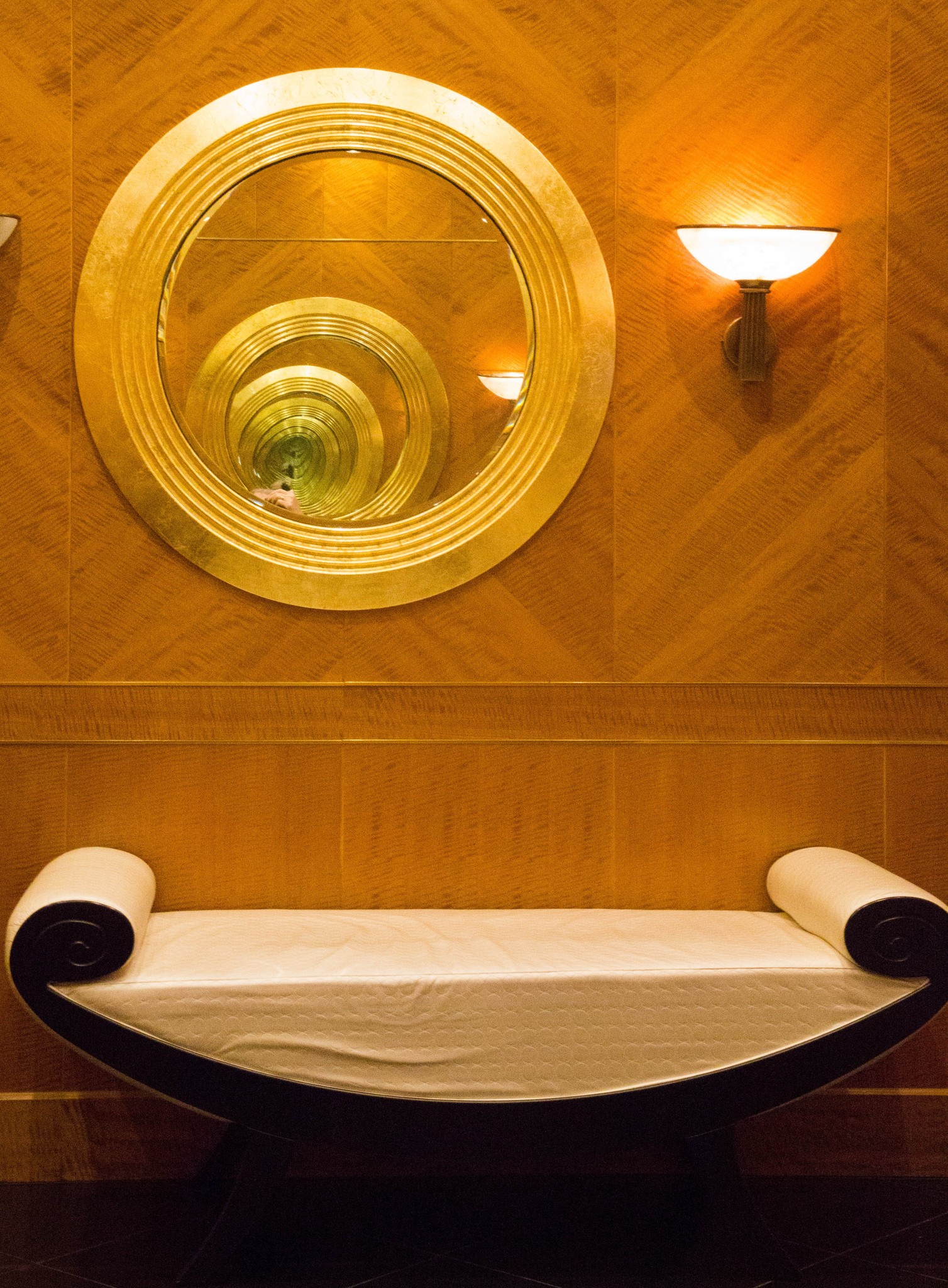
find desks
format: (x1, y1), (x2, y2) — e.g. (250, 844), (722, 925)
(2, 845), (948, 1246)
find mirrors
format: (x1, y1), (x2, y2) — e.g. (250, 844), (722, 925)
(70, 68), (618, 617)
(176, 296), (452, 531)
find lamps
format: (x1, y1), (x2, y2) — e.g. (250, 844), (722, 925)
(479, 371), (523, 402)
(676, 219), (842, 382)
